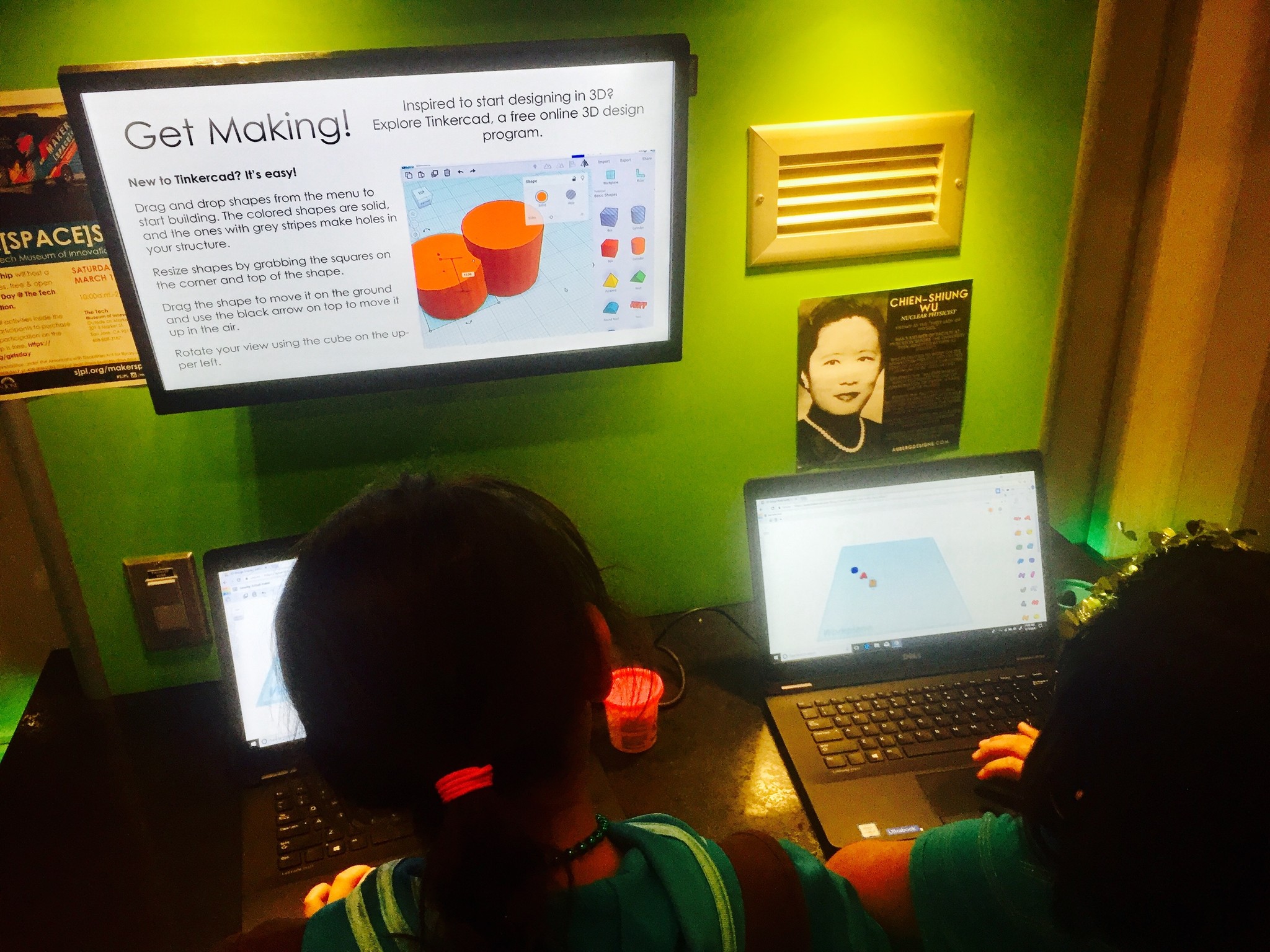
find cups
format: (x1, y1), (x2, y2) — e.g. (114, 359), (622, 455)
(604, 669), (663, 753)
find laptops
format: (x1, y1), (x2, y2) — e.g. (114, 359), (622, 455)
(740, 449), (1067, 853)
(199, 534), (629, 935)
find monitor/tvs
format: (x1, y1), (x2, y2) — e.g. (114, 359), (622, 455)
(56, 33), (690, 417)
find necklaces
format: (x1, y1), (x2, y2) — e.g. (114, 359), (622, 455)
(546, 811), (610, 865)
(802, 415), (865, 452)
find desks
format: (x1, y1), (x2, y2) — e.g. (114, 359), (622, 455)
(0, 523), (1109, 952)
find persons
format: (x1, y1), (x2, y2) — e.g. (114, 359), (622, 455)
(824, 542), (1270, 952)
(797, 295), (891, 471)
(217, 470), (888, 952)
